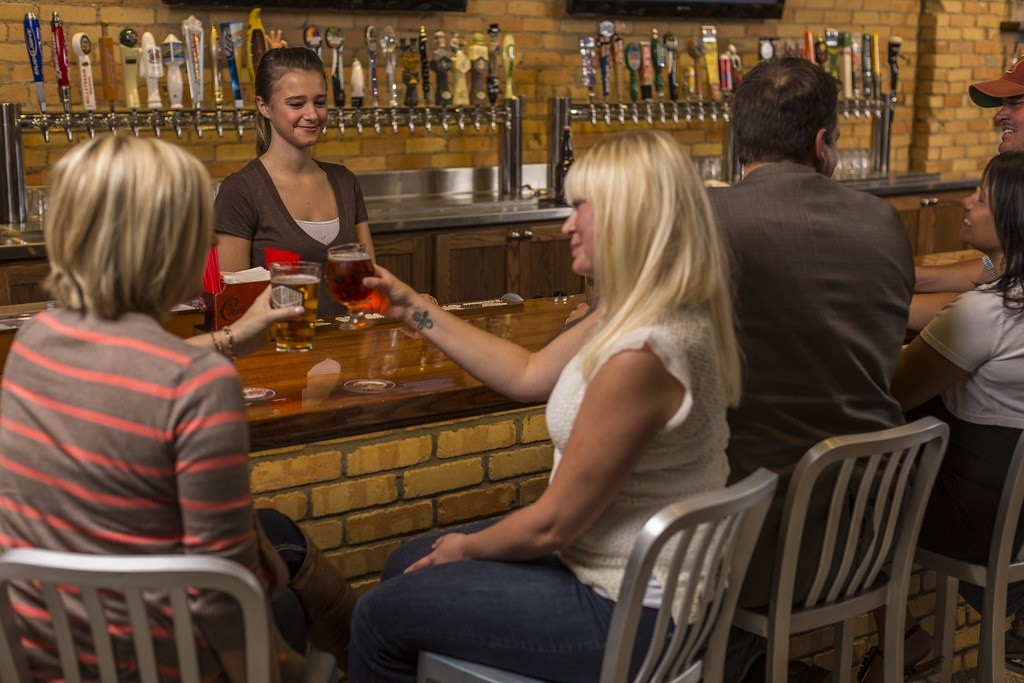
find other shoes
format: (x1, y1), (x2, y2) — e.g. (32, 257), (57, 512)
(787, 660), (834, 683)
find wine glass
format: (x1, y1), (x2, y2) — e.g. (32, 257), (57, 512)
(326, 244), (376, 330)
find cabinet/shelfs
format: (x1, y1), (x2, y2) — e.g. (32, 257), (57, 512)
(0, 187), (973, 305)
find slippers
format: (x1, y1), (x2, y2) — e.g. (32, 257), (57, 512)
(999, 618), (1024, 661)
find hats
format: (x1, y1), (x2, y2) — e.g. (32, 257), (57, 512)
(968, 57), (1024, 108)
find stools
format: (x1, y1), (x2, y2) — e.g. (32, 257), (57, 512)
(418, 467), (778, 682)
(914, 430), (1024, 683)
(1, 549), (270, 683)
(733, 418), (947, 682)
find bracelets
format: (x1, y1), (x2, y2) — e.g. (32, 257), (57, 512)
(209, 328), (224, 354)
(221, 325), (236, 363)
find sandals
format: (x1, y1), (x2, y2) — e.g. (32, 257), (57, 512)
(855, 621), (944, 683)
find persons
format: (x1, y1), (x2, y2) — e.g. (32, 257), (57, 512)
(701, 56), (1024, 683)
(0, 138), (364, 683)
(344, 128), (744, 683)
(216, 47), (378, 320)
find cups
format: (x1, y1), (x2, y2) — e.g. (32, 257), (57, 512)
(268, 261), (322, 354)
(691, 148), (875, 183)
(22, 185), (49, 227)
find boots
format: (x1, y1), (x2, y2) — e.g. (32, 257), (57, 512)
(291, 525), (356, 674)
